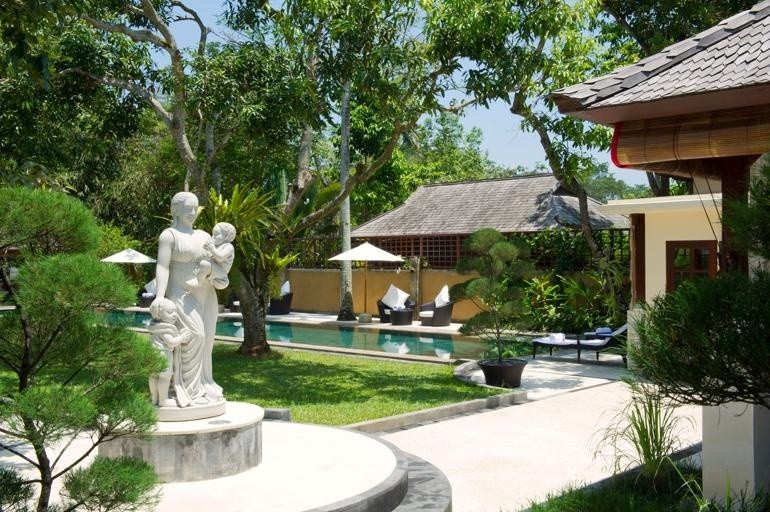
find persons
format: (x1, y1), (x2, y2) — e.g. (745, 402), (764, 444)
(142, 188), (239, 412)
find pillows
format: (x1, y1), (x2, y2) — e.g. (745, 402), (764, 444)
(280, 279), (291, 295)
(381, 283), (410, 309)
(434, 285), (450, 308)
(144, 278), (157, 294)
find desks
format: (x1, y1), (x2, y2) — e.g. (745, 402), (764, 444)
(390, 308), (414, 325)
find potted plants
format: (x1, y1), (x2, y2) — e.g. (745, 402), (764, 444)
(448, 227), (536, 388)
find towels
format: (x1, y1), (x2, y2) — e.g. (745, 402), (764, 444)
(549, 332), (565, 341)
(596, 326), (611, 333)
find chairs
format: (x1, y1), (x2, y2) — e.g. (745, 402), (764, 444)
(584, 331), (613, 337)
(377, 299), (415, 323)
(270, 292), (293, 315)
(532, 322), (628, 361)
(136, 287), (156, 308)
(420, 301), (454, 326)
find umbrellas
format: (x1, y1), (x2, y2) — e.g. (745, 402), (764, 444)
(98, 245), (158, 268)
(326, 240), (406, 313)
